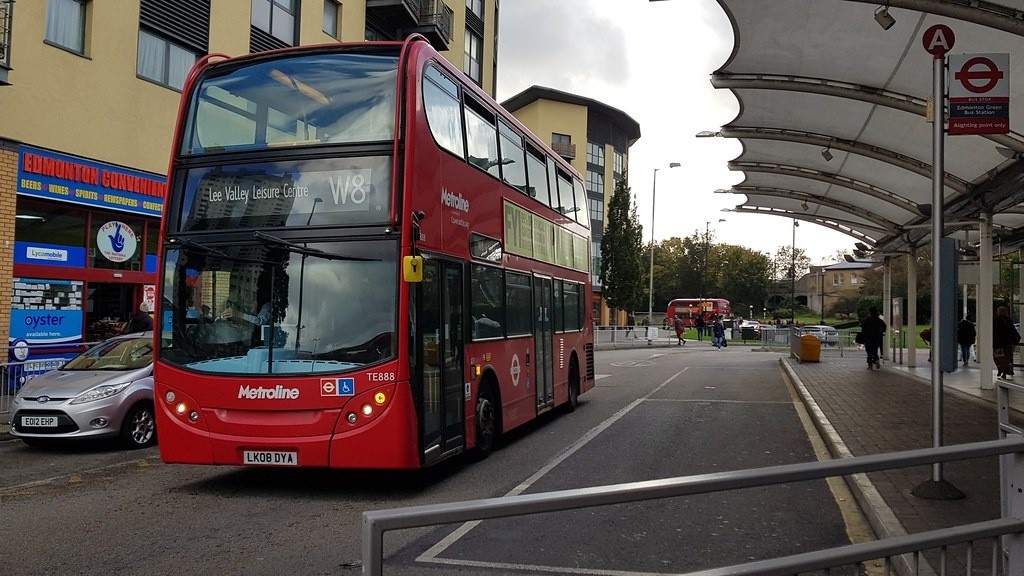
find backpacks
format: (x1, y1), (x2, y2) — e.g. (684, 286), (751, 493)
(920, 330), (931, 345)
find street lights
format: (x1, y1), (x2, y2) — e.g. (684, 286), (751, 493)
(649, 163), (681, 323)
(704, 219), (726, 336)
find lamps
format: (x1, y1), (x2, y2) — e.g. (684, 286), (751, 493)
(917, 204), (985, 221)
(845, 255), (888, 267)
(996, 147), (1024, 159)
(855, 243), (911, 253)
(802, 200), (809, 211)
(822, 147), (833, 161)
(874, 1), (896, 30)
(853, 250), (875, 258)
(794, 219), (799, 227)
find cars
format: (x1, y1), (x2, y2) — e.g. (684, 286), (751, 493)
(738, 320), (775, 341)
(7, 331), (174, 450)
(799, 325), (839, 346)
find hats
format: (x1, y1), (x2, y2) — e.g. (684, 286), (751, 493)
(998, 307), (1008, 316)
(139, 301), (149, 312)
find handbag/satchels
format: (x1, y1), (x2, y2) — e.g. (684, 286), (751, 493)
(855, 332), (864, 344)
(721, 337), (727, 347)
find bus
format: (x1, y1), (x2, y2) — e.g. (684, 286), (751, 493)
(667, 298), (732, 328)
(154, 33), (596, 469)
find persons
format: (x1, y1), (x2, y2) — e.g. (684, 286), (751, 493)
(877, 309), (886, 365)
(625, 314), (638, 338)
(776, 318), (792, 325)
(714, 320), (724, 350)
(128, 303), (154, 332)
(694, 315), (704, 341)
(221, 290), (294, 325)
(767, 320), (772, 325)
(667, 318), (673, 330)
(993, 306), (1021, 381)
(957, 314), (977, 366)
(674, 315), (686, 345)
(708, 313), (743, 330)
(644, 315), (651, 337)
(662, 318), (669, 330)
(860, 307), (888, 370)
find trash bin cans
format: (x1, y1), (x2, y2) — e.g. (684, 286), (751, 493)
(732, 328), (740, 339)
(799, 334), (821, 363)
(741, 326), (755, 340)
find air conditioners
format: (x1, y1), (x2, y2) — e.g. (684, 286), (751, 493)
(419, 0), (451, 44)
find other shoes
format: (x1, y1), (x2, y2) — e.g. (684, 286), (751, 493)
(626, 332), (628, 337)
(928, 359), (931, 362)
(712, 343), (716, 346)
(635, 336), (638, 338)
(880, 356), (885, 364)
(997, 372), (1001, 376)
(867, 366), (873, 370)
(678, 343), (681, 345)
(963, 363), (967, 366)
(717, 349), (721, 351)
(876, 361), (880, 369)
(683, 341), (686, 344)
(1001, 375), (1007, 379)
